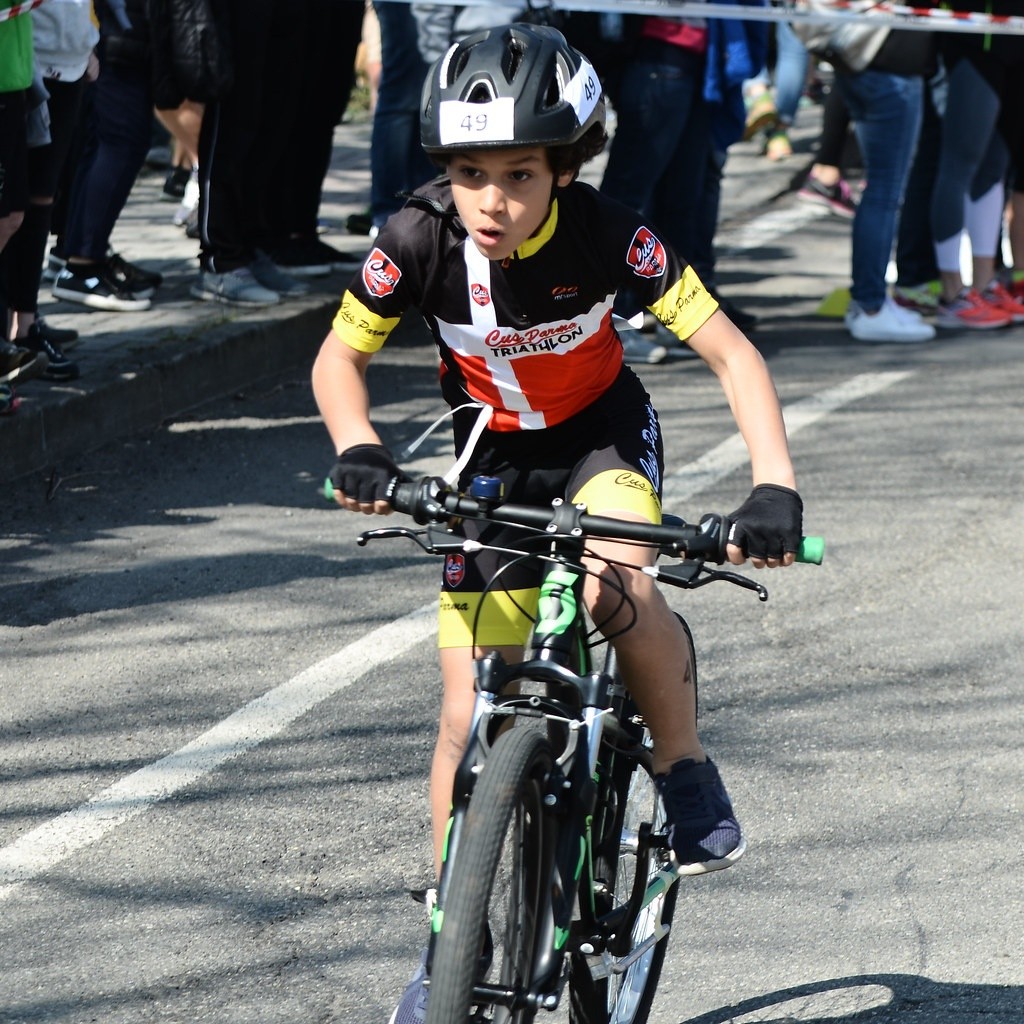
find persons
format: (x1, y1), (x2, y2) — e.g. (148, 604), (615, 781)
(0, 0), (1024, 384)
(306, 21), (805, 1024)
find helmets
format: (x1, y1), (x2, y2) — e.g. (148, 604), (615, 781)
(420, 23), (605, 158)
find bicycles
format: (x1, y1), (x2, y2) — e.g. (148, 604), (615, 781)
(319, 466), (829, 1023)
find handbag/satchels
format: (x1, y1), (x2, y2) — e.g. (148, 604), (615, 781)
(792, 0), (902, 73)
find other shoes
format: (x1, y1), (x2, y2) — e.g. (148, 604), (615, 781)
(345, 211), (377, 235)
(163, 163), (192, 198)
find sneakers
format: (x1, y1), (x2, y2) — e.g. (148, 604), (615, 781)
(654, 753), (745, 876)
(1009, 269), (1024, 305)
(978, 280), (1024, 321)
(264, 241), (331, 272)
(51, 262), (152, 311)
(292, 231), (363, 271)
(24, 318), (78, 349)
(389, 921), (493, 1024)
(934, 284), (1009, 328)
(626, 291), (757, 355)
(745, 93), (778, 139)
(893, 279), (961, 328)
(8, 322), (80, 383)
(796, 174), (857, 219)
(40, 245), (162, 297)
(763, 133), (792, 161)
(846, 296), (935, 342)
(252, 260), (311, 295)
(188, 264), (281, 307)
(0, 338), (46, 383)
